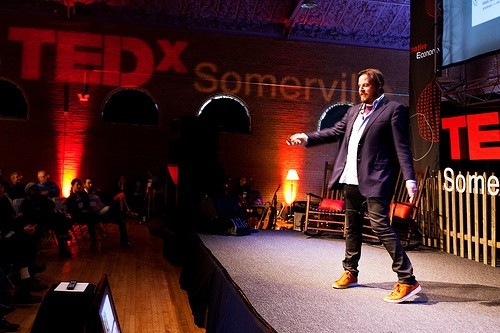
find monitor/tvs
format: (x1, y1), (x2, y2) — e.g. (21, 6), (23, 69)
(93, 272), (121, 333)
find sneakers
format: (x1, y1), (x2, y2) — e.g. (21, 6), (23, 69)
(332, 270), (358, 288)
(383, 279), (421, 302)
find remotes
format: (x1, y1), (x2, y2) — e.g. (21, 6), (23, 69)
(67, 280), (78, 289)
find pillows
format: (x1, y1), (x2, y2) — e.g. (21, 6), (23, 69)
(317, 198), (345, 211)
(388, 202), (414, 218)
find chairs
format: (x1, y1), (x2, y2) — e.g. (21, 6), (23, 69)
(2, 195), (113, 290)
(305, 161), (347, 238)
(362, 165), (431, 248)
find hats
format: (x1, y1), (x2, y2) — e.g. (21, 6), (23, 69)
(24, 182), (40, 193)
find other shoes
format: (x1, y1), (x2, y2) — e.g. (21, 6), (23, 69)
(34, 263), (46, 272)
(1, 305), (16, 315)
(26, 282), (49, 292)
(120, 243), (134, 247)
(61, 249), (73, 258)
(102, 232), (110, 239)
(20, 277), (42, 288)
(0, 317), (20, 328)
(14, 289), (44, 304)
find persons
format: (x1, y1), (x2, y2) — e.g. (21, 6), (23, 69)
(0, 168), (49, 331)
(18, 185), (74, 257)
(82, 177), (133, 248)
(232, 176), (250, 196)
(286, 68), (422, 302)
(36, 170), (60, 197)
(65, 178), (109, 253)
(112, 175), (134, 214)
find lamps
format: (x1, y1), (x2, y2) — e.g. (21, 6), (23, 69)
(285, 168), (300, 224)
(300, 0), (318, 9)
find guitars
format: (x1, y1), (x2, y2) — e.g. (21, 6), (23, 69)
(262, 184), (280, 230)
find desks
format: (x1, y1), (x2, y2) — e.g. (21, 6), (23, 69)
(29, 283), (96, 333)
